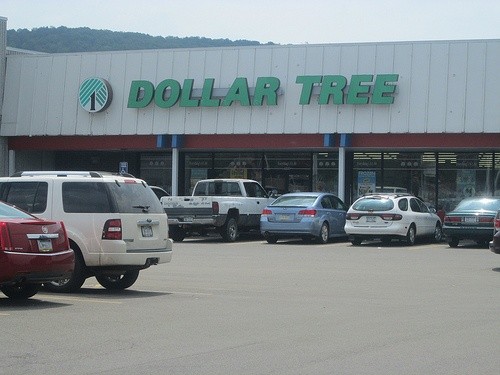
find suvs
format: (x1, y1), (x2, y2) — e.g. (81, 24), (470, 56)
(0, 170), (174, 292)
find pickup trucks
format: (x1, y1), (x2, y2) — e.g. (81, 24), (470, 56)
(158, 178), (282, 242)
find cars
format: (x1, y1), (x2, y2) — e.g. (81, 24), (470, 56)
(343, 192), (443, 246)
(441, 196), (500, 250)
(258, 191), (349, 244)
(0, 199), (76, 301)
